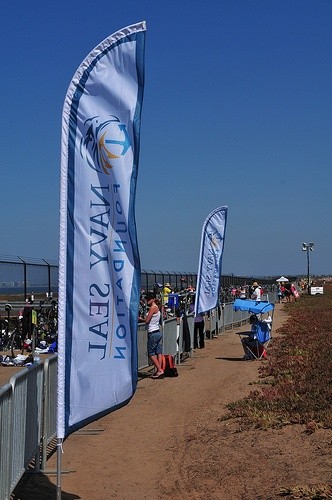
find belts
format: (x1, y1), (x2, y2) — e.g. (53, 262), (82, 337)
(148, 329), (160, 334)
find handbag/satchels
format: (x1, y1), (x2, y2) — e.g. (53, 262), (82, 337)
(200, 312), (205, 316)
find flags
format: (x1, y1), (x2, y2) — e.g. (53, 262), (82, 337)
(57, 21), (146, 438)
(194, 204), (229, 316)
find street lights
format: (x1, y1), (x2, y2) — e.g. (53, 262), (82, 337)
(302, 242), (314, 295)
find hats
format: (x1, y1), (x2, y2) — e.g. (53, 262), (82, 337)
(247, 315), (257, 319)
(253, 282), (259, 286)
(144, 292), (156, 299)
(164, 283), (170, 286)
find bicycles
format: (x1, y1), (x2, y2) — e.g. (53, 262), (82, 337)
(0, 319), (24, 359)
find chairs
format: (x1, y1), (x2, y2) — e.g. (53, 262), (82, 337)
(236, 320), (272, 360)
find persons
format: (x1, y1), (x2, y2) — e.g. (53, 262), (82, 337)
(241, 315), (259, 360)
(138, 282), (197, 321)
(220, 281), (308, 305)
(189, 293), (205, 349)
(138, 292), (165, 379)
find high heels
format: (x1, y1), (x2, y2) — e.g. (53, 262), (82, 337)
(149, 370), (164, 379)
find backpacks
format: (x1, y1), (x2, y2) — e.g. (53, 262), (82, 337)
(24, 355), (34, 364)
